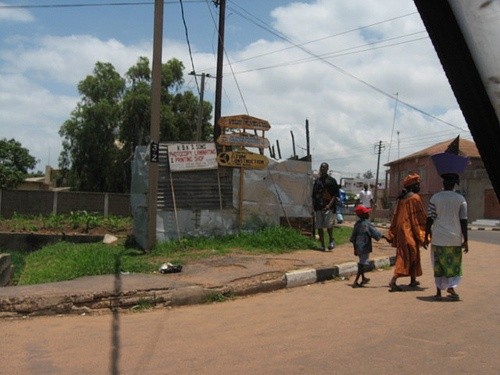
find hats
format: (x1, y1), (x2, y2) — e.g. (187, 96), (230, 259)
(354, 205), (373, 216)
(401, 173), (421, 187)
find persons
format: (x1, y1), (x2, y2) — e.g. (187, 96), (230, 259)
(312, 162), (339, 251)
(336, 184), (377, 224)
(424, 174), (469, 301)
(384, 174), (430, 292)
(350, 206), (391, 289)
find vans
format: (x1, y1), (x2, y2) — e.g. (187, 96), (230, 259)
(337, 189), (349, 202)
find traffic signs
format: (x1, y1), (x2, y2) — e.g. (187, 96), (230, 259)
(216, 115), (271, 169)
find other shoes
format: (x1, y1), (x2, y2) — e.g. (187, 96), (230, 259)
(318, 246), (325, 250)
(327, 238), (335, 249)
(433, 294), (441, 299)
(447, 292), (459, 297)
